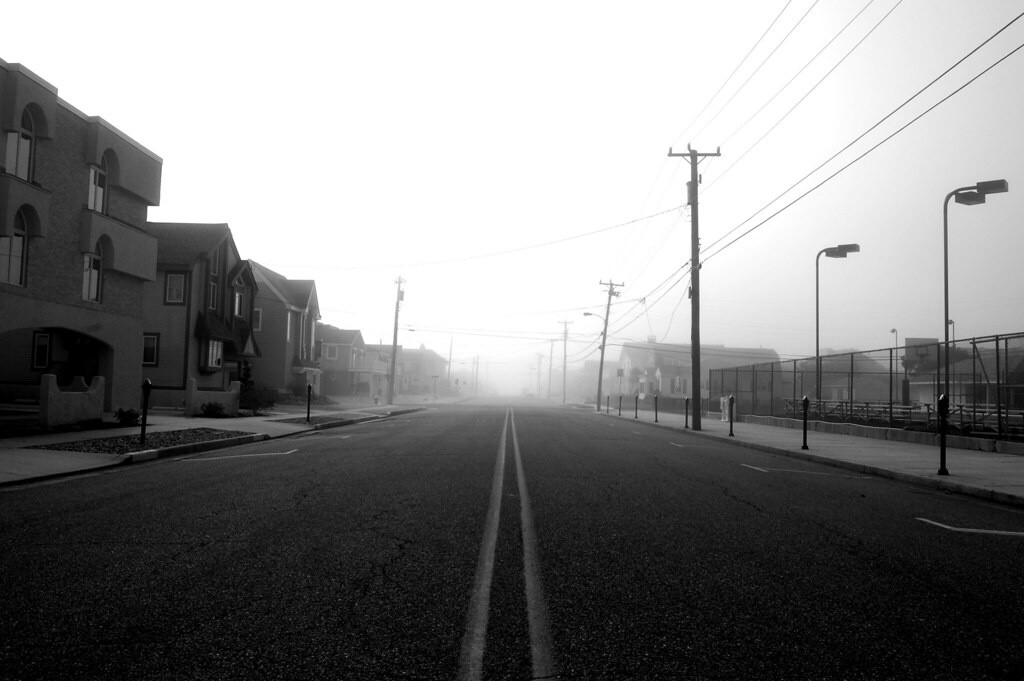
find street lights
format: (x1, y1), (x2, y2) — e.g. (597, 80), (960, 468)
(929, 178), (1010, 472)
(582, 310), (605, 412)
(811, 241), (861, 410)
(947, 316), (957, 414)
(890, 328), (900, 403)
